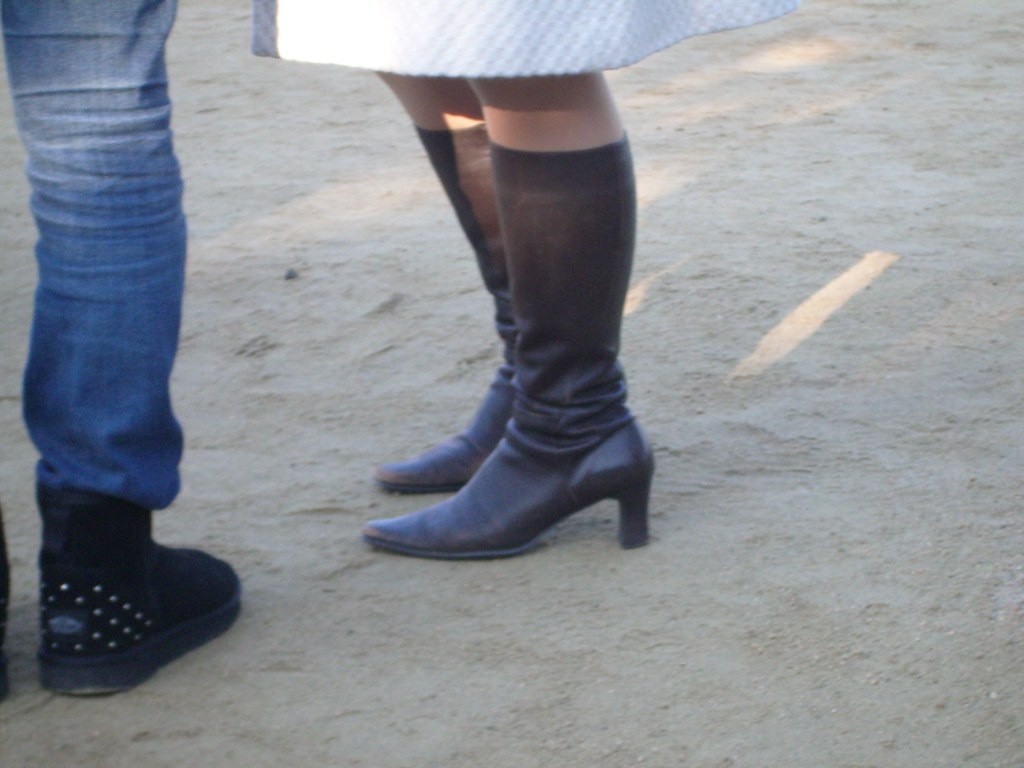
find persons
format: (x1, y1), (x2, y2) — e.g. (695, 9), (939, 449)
(250, 0), (705, 570)
(2, 4), (250, 702)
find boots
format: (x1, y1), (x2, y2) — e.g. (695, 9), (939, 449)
(360, 121), (656, 565)
(0, 474), (242, 694)
